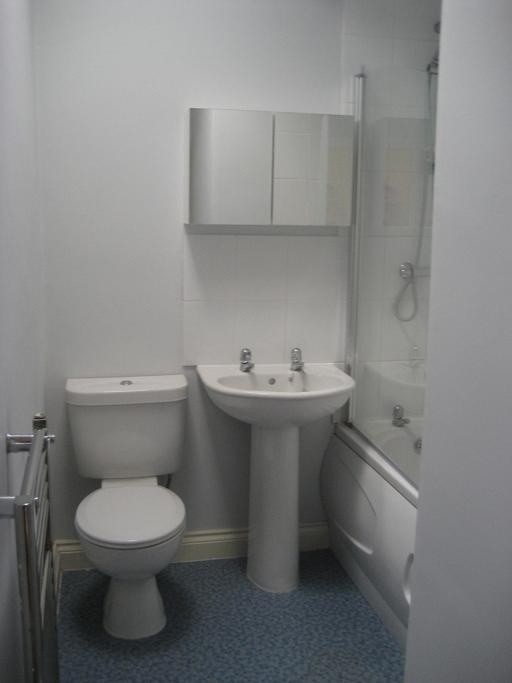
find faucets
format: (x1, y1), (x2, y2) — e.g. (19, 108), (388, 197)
(391, 404), (410, 428)
(240, 348), (255, 372)
(291, 346), (305, 371)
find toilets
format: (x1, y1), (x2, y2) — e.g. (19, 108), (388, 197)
(63, 376), (189, 640)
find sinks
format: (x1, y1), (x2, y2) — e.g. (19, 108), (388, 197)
(196, 366), (354, 420)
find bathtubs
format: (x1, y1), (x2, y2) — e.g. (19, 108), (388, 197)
(317, 418), (423, 653)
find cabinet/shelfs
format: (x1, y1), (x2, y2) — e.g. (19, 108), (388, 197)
(184, 108), (354, 236)
